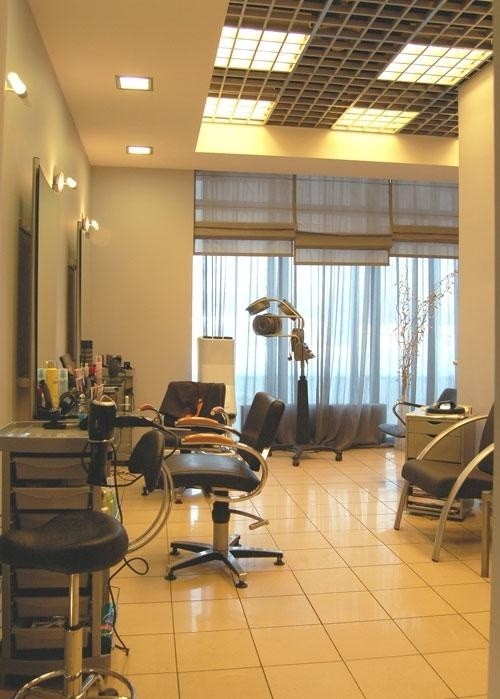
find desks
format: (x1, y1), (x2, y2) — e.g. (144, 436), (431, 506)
(479, 490), (496, 578)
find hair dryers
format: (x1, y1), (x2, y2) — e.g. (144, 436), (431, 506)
(87, 395), (155, 487)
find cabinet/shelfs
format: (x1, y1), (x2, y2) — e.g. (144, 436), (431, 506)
(0, 421), (119, 691)
(404, 402), (476, 521)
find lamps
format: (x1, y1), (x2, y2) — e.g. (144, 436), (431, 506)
(4, 70), (31, 100)
(79, 213), (100, 238)
(53, 171), (77, 193)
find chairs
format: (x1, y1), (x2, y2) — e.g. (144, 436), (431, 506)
(138, 382), (227, 454)
(376, 388), (456, 438)
(394, 402), (496, 562)
(129, 392), (285, 588)
(0, 430), (176, 699)
